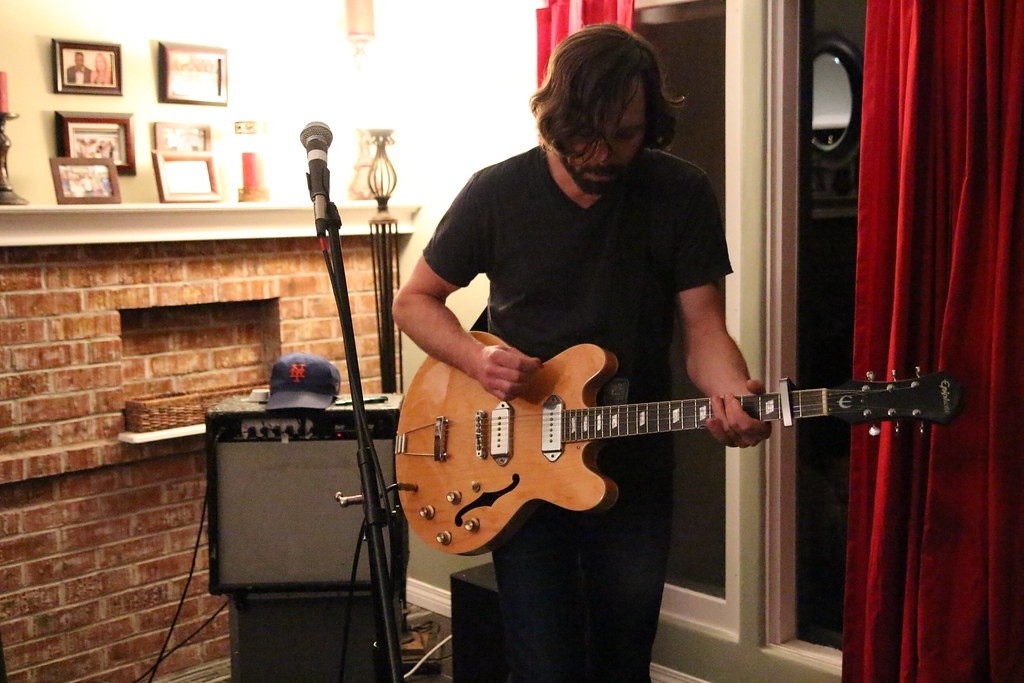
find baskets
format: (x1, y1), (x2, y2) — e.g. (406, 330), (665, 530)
(125, 381), (270, 432)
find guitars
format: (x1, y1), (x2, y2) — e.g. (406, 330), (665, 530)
(393, 330), (965, 555)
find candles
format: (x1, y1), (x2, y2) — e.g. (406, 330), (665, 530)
(240, 151), (258, 187)
(0, 72), (9, 114)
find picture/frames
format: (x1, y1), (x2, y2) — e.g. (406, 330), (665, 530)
(55, 110), (137, 176)
(152, 150), (223, 203)
(52, 38), (123, 96)
(154, 122), (211, 153)
(50, 156), (122, 204)
(158, 41), (228, 107)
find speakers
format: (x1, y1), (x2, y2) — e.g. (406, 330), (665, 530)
(204, 393), (405, 595)
(226, 587), (405, 683)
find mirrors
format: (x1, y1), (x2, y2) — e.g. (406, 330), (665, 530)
(809, 32), (862, 169)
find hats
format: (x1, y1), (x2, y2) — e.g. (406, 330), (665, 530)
(267, 352), (342, 409)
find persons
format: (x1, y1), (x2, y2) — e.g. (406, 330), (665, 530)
(90, 54), (112, 84)
(77, 139), (114, 157)
(67, 52), (91, 84)
(62, 169), (112, 196)
(391, 23), (773, 682)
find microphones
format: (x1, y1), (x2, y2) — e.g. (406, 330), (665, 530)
(300, 122), (333, 239)
